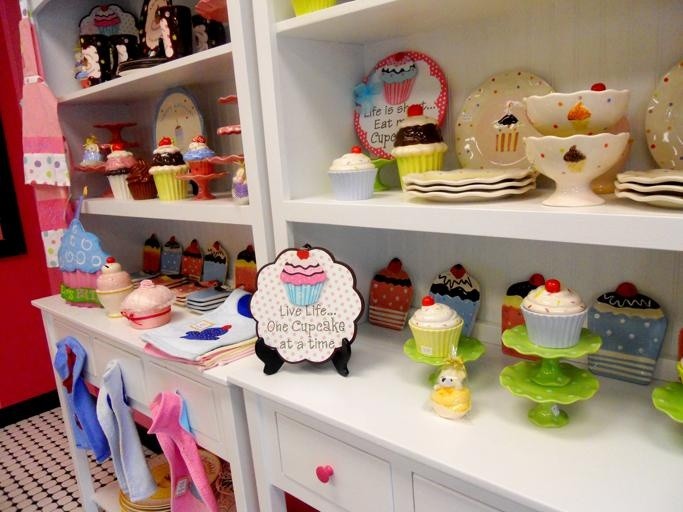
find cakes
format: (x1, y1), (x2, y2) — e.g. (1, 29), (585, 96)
(120, 280), (173, 330)
(279, 247), (326, 305)
(587, 283), (667, 384)
(409, 297), (464, 358)
(141, 232), (257, 290)
(327, 146), (378, 201)
(104, 136), (217, 200)
(58, 218), (108, 308)
(428, 264), (481, 335)
(368, 258), (413, 331)
(520, 278), (586, 349)
(95, 256), (133, 316)
(501, 272), (543, 361)
(392, 105), (448, 192)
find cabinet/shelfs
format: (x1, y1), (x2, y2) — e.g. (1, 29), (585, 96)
(20, 0), (683, 512)
